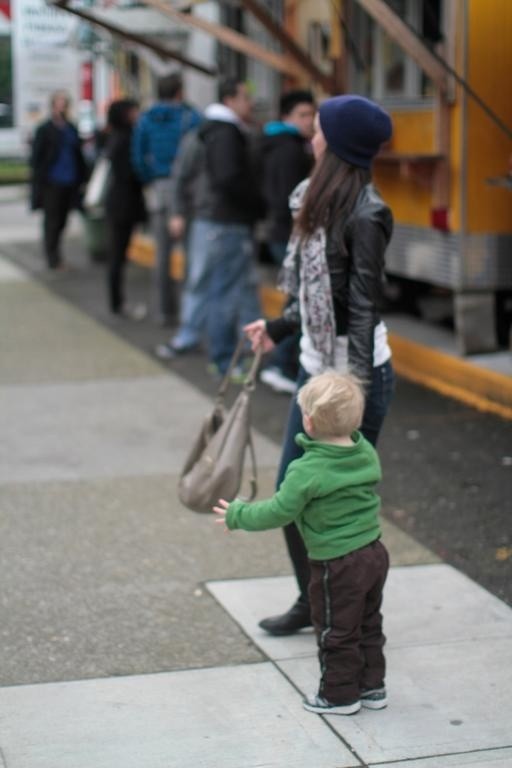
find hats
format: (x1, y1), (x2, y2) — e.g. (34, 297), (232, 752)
(319, 95), (392, 166)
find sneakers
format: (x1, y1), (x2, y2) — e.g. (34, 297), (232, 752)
(302, 686), (388, 716)
(258, 601), (315, 635)
(211, 359), (243, 384)
(153, 338), (195, 360)
(121, 302), (147, 321)
(259, 365), (297, 394)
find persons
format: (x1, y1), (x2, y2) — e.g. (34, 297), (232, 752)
(94, 98), (150, 321)
(29, 88), (94, 270)
(131, 71), (204, 327)
(256, 89), (318, 268)
(154, 128), (213, 357)
(199, 74), (263, 384)
(243, 94), (400, 636)
(213, 372), (390, 716)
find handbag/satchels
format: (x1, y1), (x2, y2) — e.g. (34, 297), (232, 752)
(82, 156), (114, 219)
(176, 393), (257, 514)
